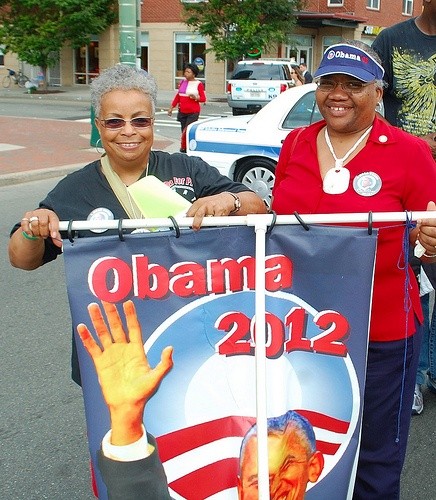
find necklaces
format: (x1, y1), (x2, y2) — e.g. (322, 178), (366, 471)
(323, 125), (373, 195)
(111, 162), (150, 233)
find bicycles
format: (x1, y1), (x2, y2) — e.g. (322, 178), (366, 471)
(2, 68), (30, 88)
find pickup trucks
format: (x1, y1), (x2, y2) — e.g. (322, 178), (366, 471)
(224, 58), (303, 116)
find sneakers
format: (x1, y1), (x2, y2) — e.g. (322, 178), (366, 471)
(410, 385), (425, 417)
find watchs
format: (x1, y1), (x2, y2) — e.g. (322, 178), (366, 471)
(223, 191), (240, 216)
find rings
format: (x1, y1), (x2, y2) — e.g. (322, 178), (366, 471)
(22, 217), (29, 221)
(29, 217), (39, 221)
(434, 246), (436, 248)
(206, 215), (213, 217)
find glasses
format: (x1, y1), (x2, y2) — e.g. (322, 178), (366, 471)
(315, 79), (377, 93)
(100, 116), (154, 128)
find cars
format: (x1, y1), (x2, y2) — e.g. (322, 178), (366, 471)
(184, 83), (384, 214)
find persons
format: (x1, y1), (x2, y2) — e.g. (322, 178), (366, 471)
(273, 42), (436, 500)
(76, 299), (324, 500)
(298, 63), (313, 84)
(167, 63), (207, 152)
(290, 67), (304, 86)
(369, 0), (436, 415)
(7, 63), (267, 496)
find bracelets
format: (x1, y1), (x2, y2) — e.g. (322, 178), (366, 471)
(413, 238), (436, 258)
(22, 230), (43, 240)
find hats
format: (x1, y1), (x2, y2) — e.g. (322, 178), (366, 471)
(312, 43), (386, 83)
